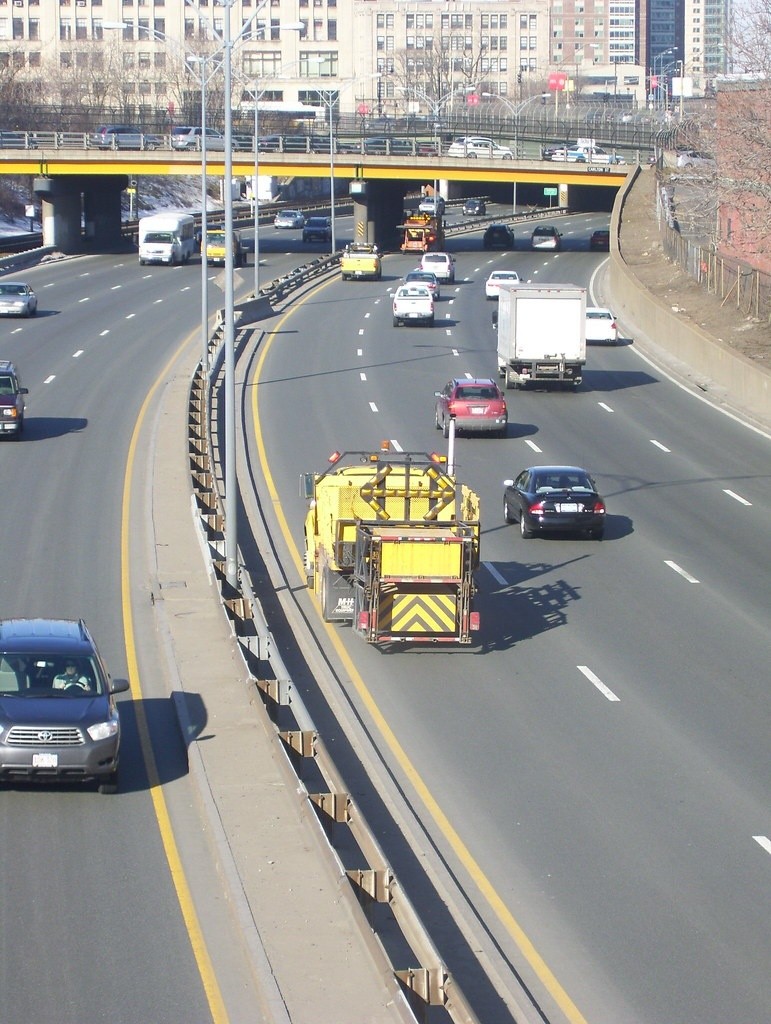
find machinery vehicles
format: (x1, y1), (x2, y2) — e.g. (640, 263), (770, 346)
(396, 212), (446, 255)
(298, 413), (481, 644)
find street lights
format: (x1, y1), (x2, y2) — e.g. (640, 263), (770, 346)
(187, 56), (325, 295)
(555, 43), (599, 136)
(394, 87), (476, 216)
(482, 92), (551, 213)
(654, 47), (680, 113)
(278, 73), (382, 253)
(103, 22), (304, 371)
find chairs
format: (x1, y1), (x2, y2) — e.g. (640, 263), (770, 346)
(0, 656), (30, 693)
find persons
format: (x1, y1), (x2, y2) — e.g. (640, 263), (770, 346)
(52, 659), (91, 692)
(456, 387), (464, 399)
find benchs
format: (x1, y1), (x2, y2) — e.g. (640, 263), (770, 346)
(536, 486), (591, 493)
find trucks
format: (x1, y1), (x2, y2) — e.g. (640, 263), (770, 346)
(138, 213), (194, 266)
(200, 230), (250, 267)
(492, 283), (587, 389)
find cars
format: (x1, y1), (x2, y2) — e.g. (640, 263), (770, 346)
(339, 196), (619, 540)
(274, 210), (331, 242)
(0, 617), (130, 794)
(0, 281), (37, 318)
(0, 126), (625, 165)
(0, 360), (28, 441)
(197, 224), (225, 244)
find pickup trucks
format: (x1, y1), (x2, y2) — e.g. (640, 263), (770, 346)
(339, 242), (383, 281)
